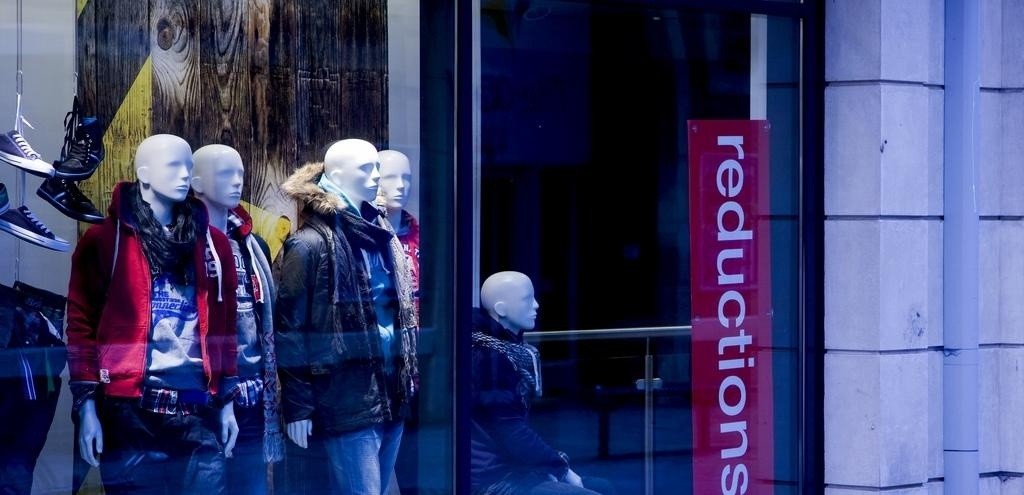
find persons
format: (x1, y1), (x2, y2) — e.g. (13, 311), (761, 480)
(190, 144), (287, 493)
(273, 136), (424, 495)
(57, 133), (243, 495)
(457, 271), (614, 495)
(375, 149), (421, 412)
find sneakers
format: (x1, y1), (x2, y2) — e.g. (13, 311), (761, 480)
(55, 95), (105, 181)
(0, 204), (72, 252)
(36, 160), (105, 223)
(0, 93), (56, 180)
